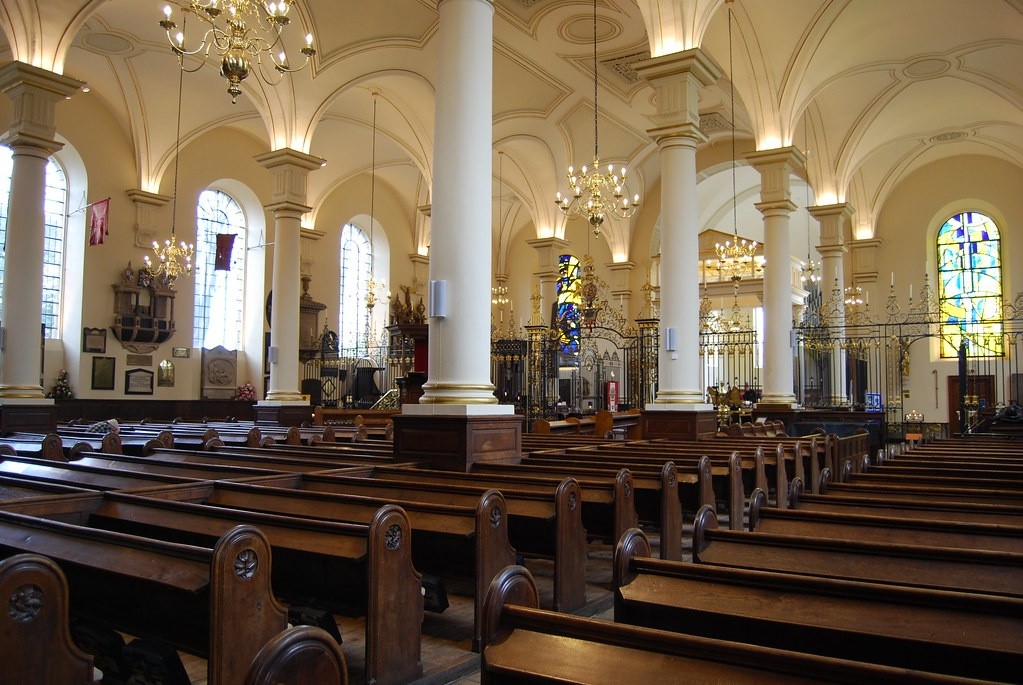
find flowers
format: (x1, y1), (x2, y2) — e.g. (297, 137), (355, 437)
(237, 380), (259, 402)
(46, 369), (75, 401)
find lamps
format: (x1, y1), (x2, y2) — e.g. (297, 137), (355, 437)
(140, 6), (195, 290)
(552, 0), (642, 240)
(362, 84), (388, 313)
(157, 0), (318, 107)
(700, 0), (759, 336)
(491, 152), (523, 330)
(795, 107), (933, 309)
(620, 294), (624, 307)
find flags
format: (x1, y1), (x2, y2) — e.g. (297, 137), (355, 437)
(215, 233), (237, 270)
(89, 199), (109, 247)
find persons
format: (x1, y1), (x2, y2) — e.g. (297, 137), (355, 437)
(740, 382), (757, 403)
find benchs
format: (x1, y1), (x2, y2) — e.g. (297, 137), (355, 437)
(1, 419), (1023, 685)
